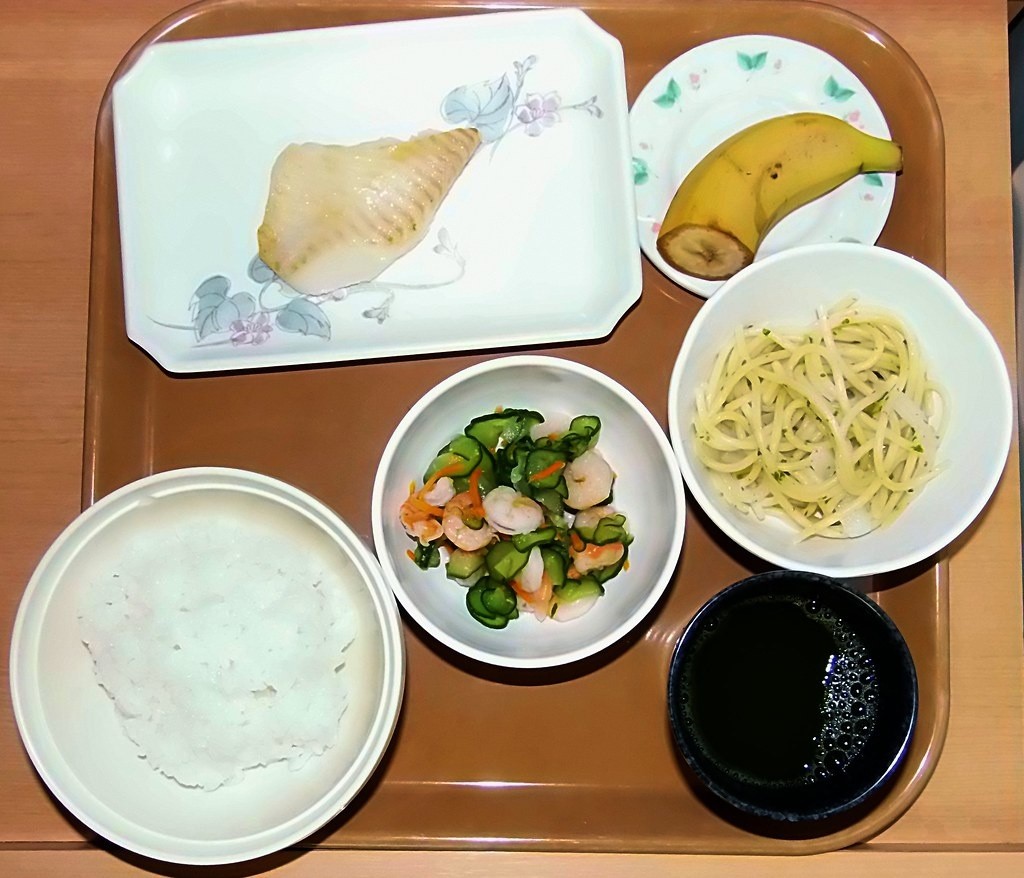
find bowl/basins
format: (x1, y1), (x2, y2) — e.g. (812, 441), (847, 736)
(370, 352), (687, 672)
(669, 242), (1014, 578)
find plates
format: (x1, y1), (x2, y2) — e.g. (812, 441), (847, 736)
(5, 463), (407, 869)
(630, 33), (897, 300)
(664, 570), (921, 842)
(110, 4), (646, 377)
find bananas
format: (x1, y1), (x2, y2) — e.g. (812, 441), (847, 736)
(657, 112), (903, 281)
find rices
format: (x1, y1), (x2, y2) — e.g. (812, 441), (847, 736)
(75, 522), (359, 792)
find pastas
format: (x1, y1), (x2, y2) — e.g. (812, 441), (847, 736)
(694, 295), (949, 545)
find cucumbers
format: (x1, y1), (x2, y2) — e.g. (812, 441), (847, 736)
(410, 409), (632, 629)
